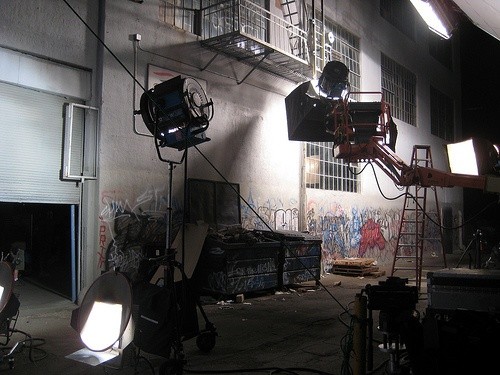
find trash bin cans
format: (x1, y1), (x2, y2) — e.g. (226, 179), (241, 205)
(254, 230), (325, 290)
(206, 232), (282, 300)
(425, 269), (500, 316)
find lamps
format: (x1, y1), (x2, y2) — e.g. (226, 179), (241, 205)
(444, 134), (500, 179)
(408, 0), (460, 41)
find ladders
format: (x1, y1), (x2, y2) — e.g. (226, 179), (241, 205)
(390, 143), (449, 295)
